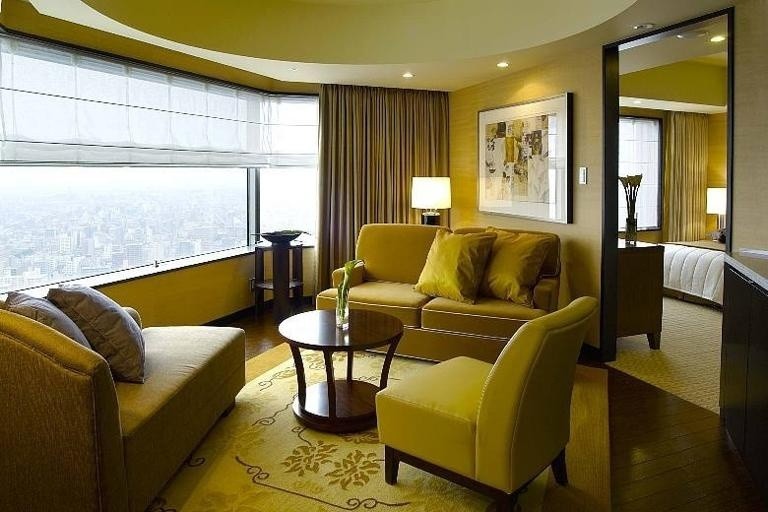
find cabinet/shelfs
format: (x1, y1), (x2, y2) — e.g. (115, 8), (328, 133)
(253, 241), (305, 325)
(617, 238), (665, 351)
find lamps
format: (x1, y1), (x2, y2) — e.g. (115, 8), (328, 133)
(705, 188), (727, 230)
(410, 176), (452, 225)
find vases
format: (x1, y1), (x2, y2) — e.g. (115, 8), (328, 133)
(624, 212), (638, 244)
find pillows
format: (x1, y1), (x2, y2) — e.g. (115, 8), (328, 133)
(416, 228), (500, 305)
(475, 225), (558, 309)
(4, 290), (92, 349)
(47, 284), (146, 385)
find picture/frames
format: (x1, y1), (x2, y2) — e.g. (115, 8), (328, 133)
(477, 91), (573, 225)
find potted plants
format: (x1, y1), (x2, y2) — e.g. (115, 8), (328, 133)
(335, 258), (364, 328)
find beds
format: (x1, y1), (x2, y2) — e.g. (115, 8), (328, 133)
(657, 240), (725, 312)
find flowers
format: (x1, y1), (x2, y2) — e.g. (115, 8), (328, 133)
(618, 173), (643, 236)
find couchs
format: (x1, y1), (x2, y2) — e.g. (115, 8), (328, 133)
(315, 223), (562, 366)
(375, 295), (599, 509)
(1, 284), (246, 509)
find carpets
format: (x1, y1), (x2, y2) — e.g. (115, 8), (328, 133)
(145, 342), (612, 511)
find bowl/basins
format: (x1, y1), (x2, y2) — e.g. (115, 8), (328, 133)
(258, 230), (302, 246)
